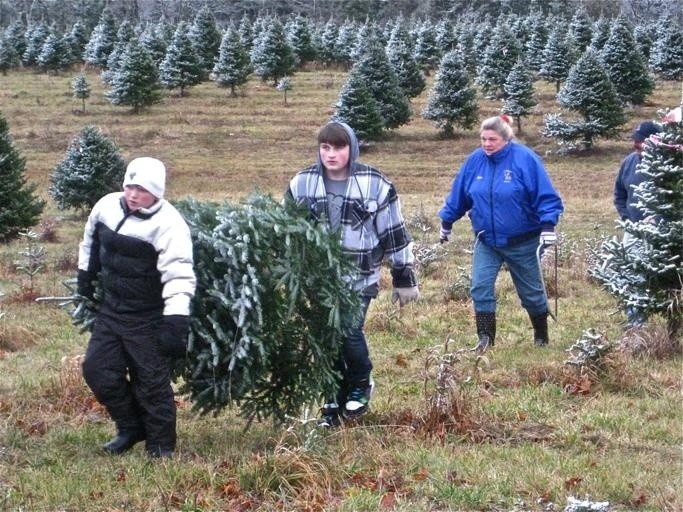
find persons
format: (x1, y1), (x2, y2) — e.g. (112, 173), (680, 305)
(611, 119), (663, 331)
(277, 119), (421, 427)
(437, 115), (563, 354)
(74, 156), (197, 460)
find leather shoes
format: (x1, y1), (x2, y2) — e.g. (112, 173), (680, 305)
(102, 428), (173, 458)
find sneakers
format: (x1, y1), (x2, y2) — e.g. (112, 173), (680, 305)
(318, 367), (375, 429)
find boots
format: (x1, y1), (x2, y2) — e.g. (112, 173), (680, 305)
(529, 312), (548, 346)
(470, 312), (496, 353)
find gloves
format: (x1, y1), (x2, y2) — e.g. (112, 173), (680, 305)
(539, 225), (557, 247)
(440, 220), (452, 244)
(391, 285), (420, 307)
(77, 270), (99, 303)
(158, 316), (189, 358)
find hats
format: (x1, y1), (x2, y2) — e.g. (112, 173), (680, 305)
(630, 121), (663, 142)
(122, 157), (167, 199)
